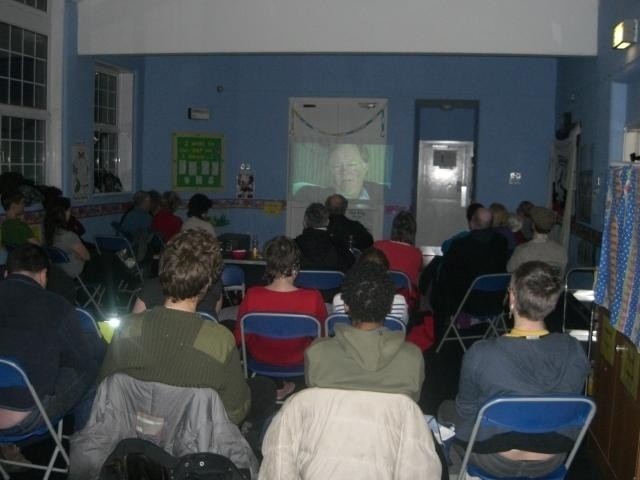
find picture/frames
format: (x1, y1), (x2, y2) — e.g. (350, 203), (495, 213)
(170, 130), (227, 194)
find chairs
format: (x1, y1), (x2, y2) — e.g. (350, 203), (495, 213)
(440, 395), (601, 479)
(48, 221), (514, 384)
(0, 354), (76, 478)
(295, 388), (411, 479)
(105, 372), (211, 479)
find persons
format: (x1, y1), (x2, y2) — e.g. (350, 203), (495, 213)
(301, 256), (426, 407)
(94, 226), (280, 440)
(0, 239), (109, 448)
(294, 139), (385, 206)
(294, 194), (431, 334)
(0, 187), (233, 323)
(437, 256), (592, 479)
(419, 201), (568, 319)
(233, 233), (329, 400)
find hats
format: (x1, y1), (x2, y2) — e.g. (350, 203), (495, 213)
(529, 206), (555, 230)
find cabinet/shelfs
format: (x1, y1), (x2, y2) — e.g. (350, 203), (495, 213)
(583, 304), (639, 479)
(561, 266), (600, 345)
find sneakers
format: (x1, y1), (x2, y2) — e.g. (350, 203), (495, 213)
(276, 381), (296, 400)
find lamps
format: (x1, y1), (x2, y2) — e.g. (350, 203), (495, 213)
(606, 15), (640, 53)
